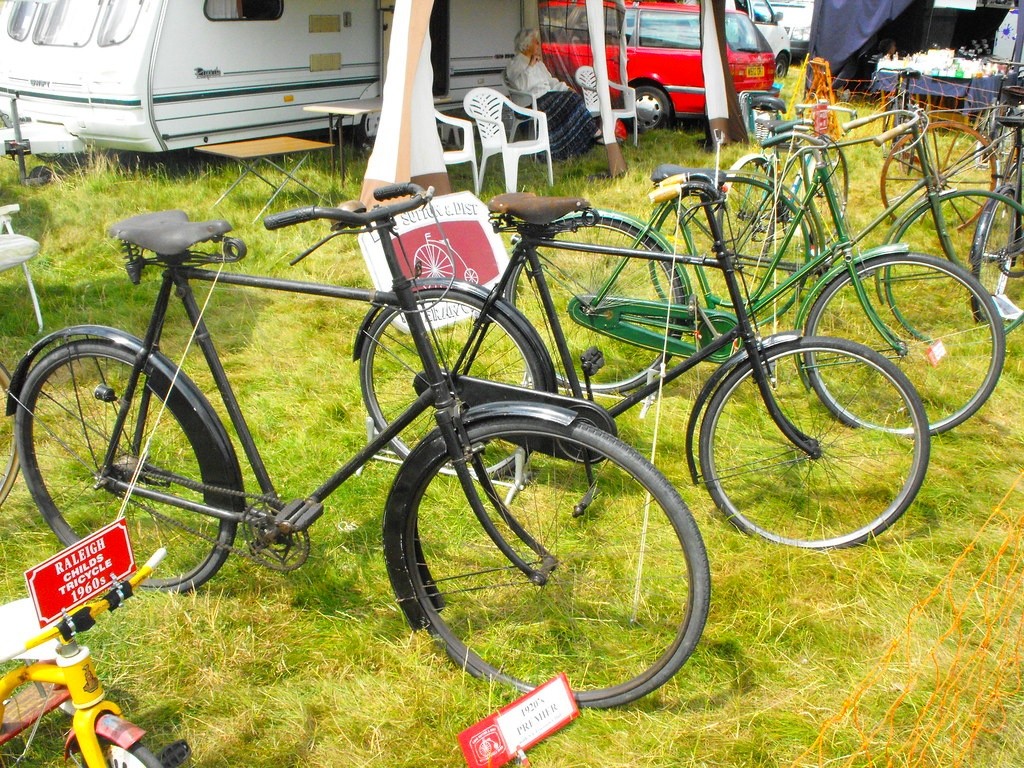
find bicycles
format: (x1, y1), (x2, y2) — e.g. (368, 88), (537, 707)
(352, 173), (934, 550)
(501, 54), (1024, 437)
(6, 180), (712, 711)
(0, 518), (195, 768)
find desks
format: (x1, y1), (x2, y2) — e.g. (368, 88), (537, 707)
(194, 137), (335, 224)
(304, 96), (382, 188)
(872, 70), (1018, 135)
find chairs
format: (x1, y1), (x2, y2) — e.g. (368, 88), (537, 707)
(433, 108), (479, 196)
(575, 66), (638, 147)
(0, 204), (44, 335)
(499, 69), (537, 164)
(463, 87), (553, 193)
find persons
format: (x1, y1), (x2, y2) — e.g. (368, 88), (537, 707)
(506, 28), (624, 164)
(877, 39), (895, 61)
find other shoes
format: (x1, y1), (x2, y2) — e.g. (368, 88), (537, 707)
(592, 132), (621, 146)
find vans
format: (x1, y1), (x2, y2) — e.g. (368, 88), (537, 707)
(537, 0), (779, 135)
(682, 0), (794, 78)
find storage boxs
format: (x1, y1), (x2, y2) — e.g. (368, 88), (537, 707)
(359, 191), (510, 335)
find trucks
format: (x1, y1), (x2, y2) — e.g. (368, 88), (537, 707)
(0, 0), (540, 154)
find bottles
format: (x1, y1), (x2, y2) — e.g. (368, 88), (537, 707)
(878, 54), (998, 77)
